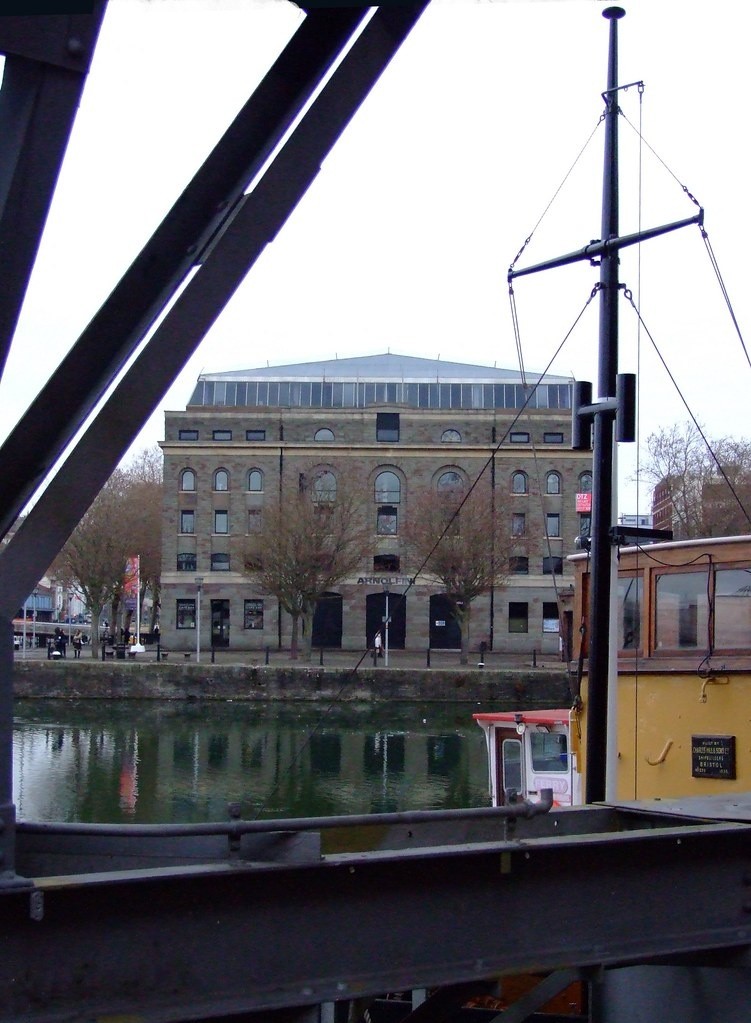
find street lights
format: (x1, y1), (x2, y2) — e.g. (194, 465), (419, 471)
(32, 588), (39, 649)
(382, 581), (391, 666)
(195, 576), (204, 660)
(67, 593), (73, 646)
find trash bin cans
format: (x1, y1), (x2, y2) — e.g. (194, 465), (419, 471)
(116, 643), (125, 659)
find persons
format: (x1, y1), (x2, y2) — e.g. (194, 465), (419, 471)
(53, 627), (67, 659)
(74, 628), (82, 658)
(122, 628), (130, 645)
(372, 630), (384, 658)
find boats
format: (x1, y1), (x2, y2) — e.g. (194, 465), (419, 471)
(474, 709), (583, 808)
